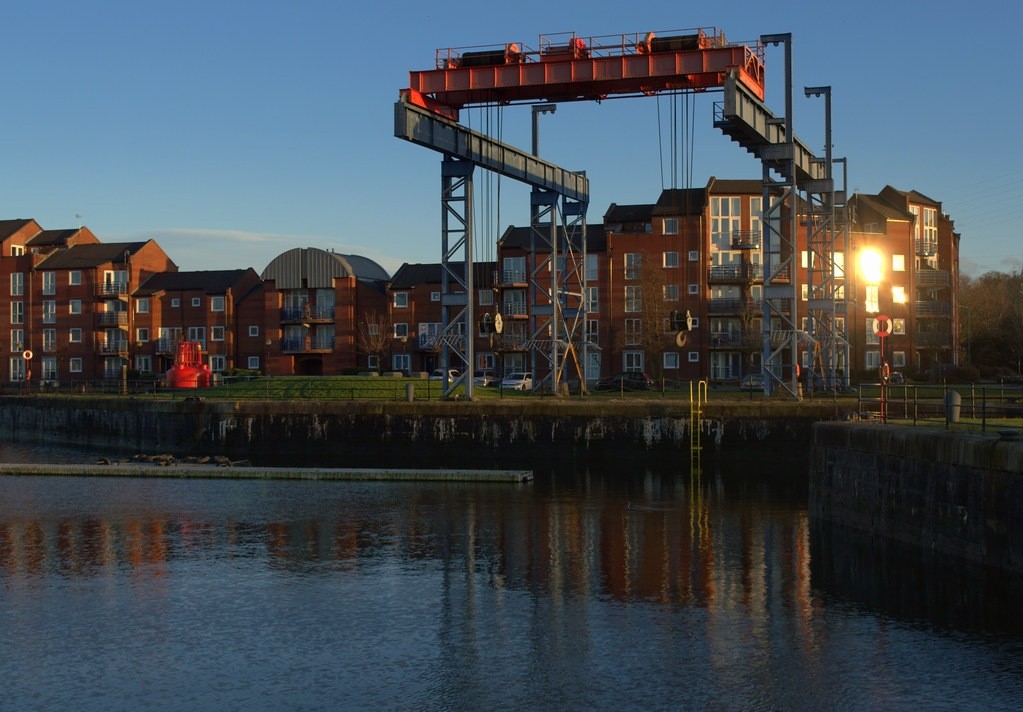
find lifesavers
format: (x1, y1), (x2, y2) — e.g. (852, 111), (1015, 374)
(879, 362), (890, 382)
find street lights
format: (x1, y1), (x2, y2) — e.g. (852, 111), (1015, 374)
(14, 342), (23, 397)
(401, 335), (409, 379)
(712, 331), (720, 389)
(265, 338), (272, 376)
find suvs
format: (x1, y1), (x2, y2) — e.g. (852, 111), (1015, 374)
(597, 370), (655, 392)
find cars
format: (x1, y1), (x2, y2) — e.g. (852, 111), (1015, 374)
(428, 367), (463, 383)
(464, 367), (503, 388)
(889, 372), (905, 384)
(740, 374), (765, 391)
(498, 372), (536, 391)
(813, 370), (845, 390)
